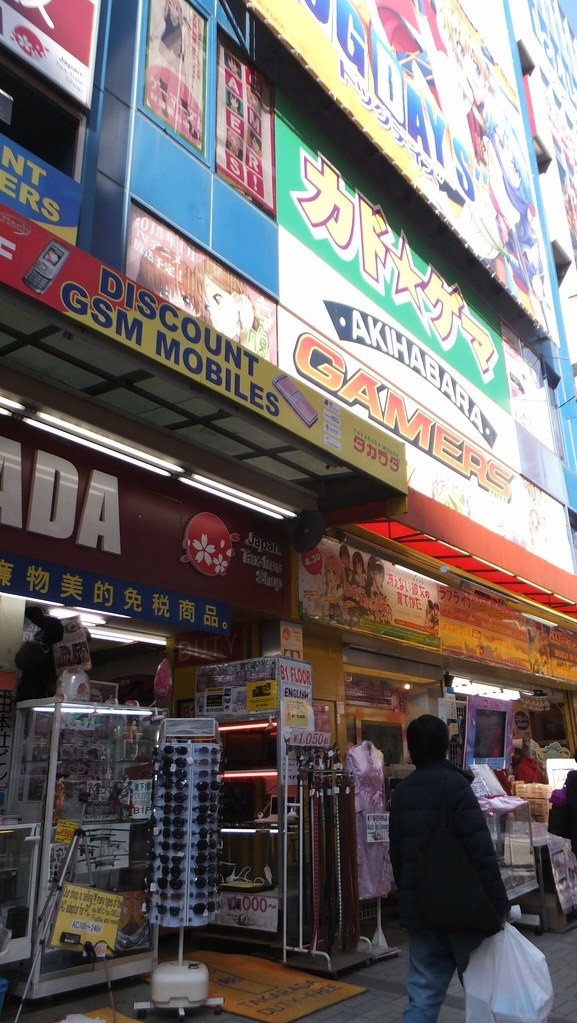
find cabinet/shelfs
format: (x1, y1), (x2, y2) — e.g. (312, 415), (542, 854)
(483, 802), (539, 901)
(6, 696), (163, 999)
(0, 822), (41, 964)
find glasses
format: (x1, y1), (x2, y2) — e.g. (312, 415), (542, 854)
(145, 745), (227, 916)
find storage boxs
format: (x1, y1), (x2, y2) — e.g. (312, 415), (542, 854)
(510, 780), (552, 822)
(519, 890), (568, 930)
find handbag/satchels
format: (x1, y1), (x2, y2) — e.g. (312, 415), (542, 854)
(462, 905), (553, 1023)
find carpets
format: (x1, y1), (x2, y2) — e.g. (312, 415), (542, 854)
(140, 949), (369, 1023)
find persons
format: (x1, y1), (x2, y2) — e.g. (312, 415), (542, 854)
(547, 771), (577, 864)
(388, 715), (512, 1023)
(125, 719), (143, 760)
(51, 775), (64, 822)
(109, 773), (135, 822)
(346, 739), (395, 901)
(512, 747), (545, 784)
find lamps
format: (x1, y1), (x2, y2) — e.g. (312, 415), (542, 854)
(0, 387), (301, 521)
